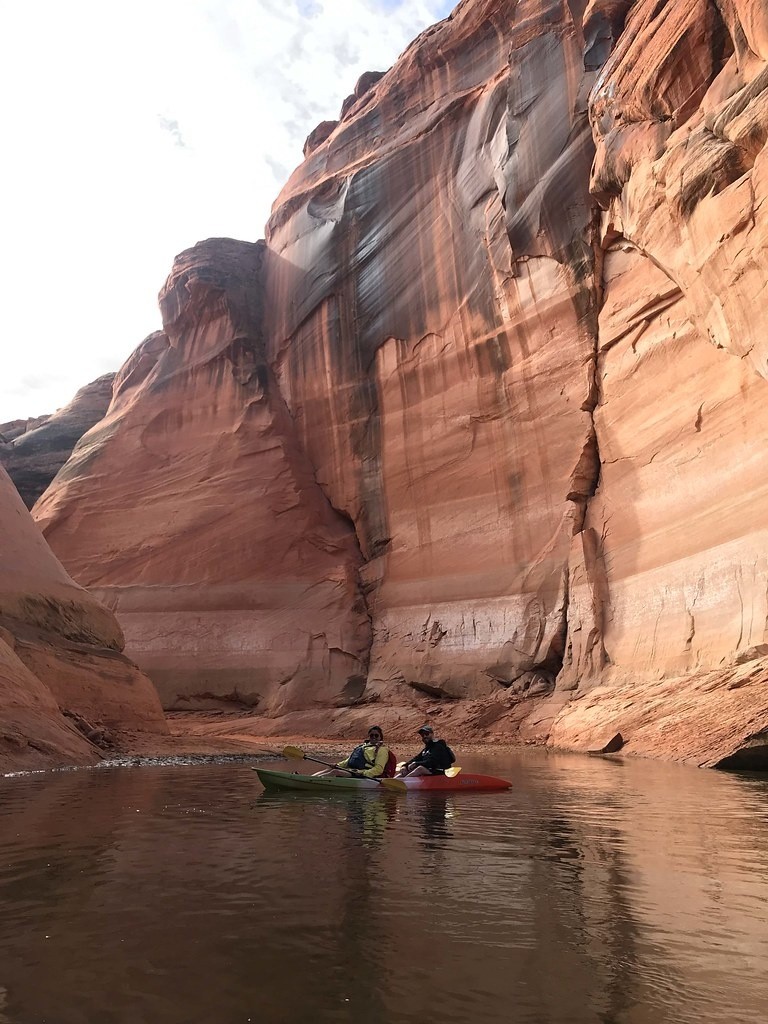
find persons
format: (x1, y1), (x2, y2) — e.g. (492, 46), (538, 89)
(393, 727), (456, 778)
(311, 724), (389, 778)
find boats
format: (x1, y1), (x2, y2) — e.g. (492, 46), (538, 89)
(249, 763), (513, 794)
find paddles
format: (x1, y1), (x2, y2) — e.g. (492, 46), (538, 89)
(282, 744), (409, 795)
(395, 761), (463, 779)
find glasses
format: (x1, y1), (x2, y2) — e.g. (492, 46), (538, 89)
(369, 734), (381, 738)
(420, 732), (429, 736)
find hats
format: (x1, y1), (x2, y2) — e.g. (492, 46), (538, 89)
(418, 726), (432, 731)
